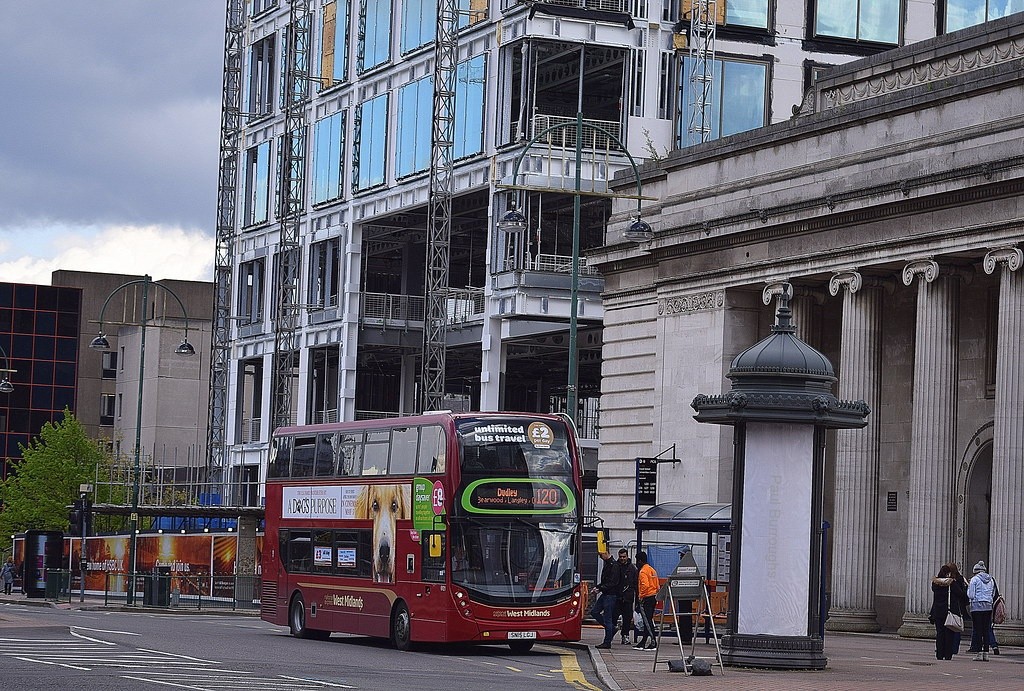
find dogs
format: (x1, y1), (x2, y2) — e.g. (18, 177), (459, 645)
(355, 484), (409, 585)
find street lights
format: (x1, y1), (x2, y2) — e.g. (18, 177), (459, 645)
(87, 273), (195, 606)
(496, 118), (653, 451)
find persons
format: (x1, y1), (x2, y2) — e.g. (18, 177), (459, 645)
(0, 556), (16, 596)
(441, 547), (469, 570)
(929, 561), (1000, 661)
(590, 541), (660, 651)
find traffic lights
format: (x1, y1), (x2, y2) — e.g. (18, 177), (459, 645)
(68, 510), (83, 536)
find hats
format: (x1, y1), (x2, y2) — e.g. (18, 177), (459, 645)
(972, 561), (986, 574)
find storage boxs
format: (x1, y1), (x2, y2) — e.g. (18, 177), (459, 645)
(261, 497), (266, 506)
(153, 517), (237, 530)
(198, 493), (220, 504)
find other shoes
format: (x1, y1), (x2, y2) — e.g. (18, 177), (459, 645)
(973, 651), (990, 662)
(632, 642), (646, 651)
(643, 645), (657, 651)
(595, 641), (611, 649)
(622, 634), (631, 645)
(611, 626), (619, 637)
(993, 649), (1000, 655)
(966, 647), (980, 653)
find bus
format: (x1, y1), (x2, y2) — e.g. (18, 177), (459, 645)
(259, 410), (609, 652)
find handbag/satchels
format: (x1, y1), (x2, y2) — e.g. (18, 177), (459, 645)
(633, 610), (645, 631)
(944, 610), (964, 633)
(991, 577), (1006, 624)
